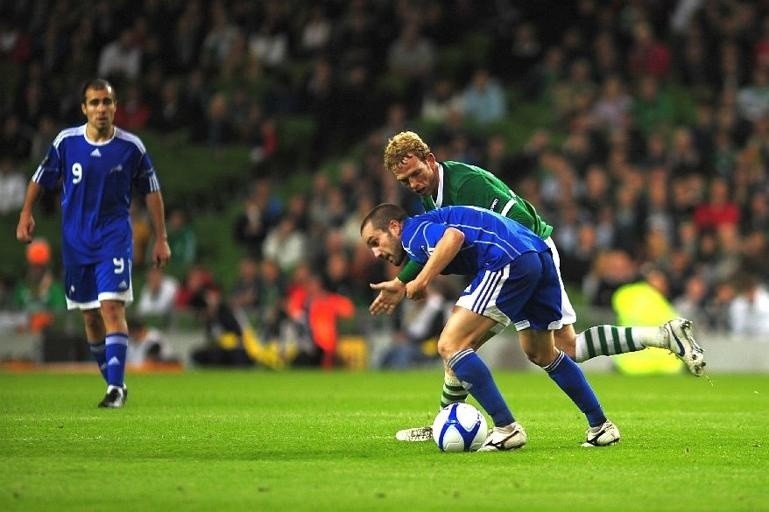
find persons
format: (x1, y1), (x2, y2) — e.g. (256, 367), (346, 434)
(362, 202), (621, 452)
(11, 238), (87, 367)
(534, 197), (623, 304)
(86, 5), (172, 146)
(130, 200), (171, 366)
(368, 130), (706, 442)
(16, 79), (171, 408)
(0, 1), (85, 174)
(174, 0), (366, 372)
(368, 273), (455, 371)
(440, 2), (767, 196)
(623, 197), (765, 334)
(369, 3), (436, 128)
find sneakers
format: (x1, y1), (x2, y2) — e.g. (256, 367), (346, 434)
(398, 423), (436, 442)
(581, 420), (619, 446)
(665, 318), (706, 376)
(476, 424), (525, 452)
(100, 385), (128, 408)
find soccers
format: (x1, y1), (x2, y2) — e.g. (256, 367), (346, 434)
(432, 403), (488, 453)
